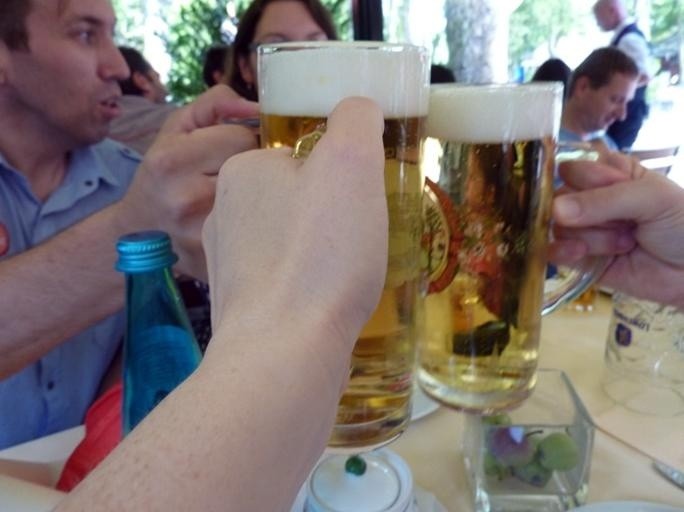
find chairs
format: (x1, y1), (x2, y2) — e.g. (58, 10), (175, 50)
(628, 147), (679, 177)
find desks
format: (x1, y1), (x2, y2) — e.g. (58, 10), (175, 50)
(0, 281), (683, 512)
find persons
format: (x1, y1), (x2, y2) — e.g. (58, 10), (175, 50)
(546, 153), (684, 309)
(98, 1), (337, 157)
(529, 1), (654, 190)
(60, 97), (390, 510)
(0, 2), (267, 455)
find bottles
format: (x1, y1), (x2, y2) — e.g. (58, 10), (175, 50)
(111, 229), (206, 445)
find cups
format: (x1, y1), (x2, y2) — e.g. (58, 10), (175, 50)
(416, 82), (618, 419)
(603, 285), (682, 412)
(306, 448), (410, 508)
(256, 40), (432, 458)
